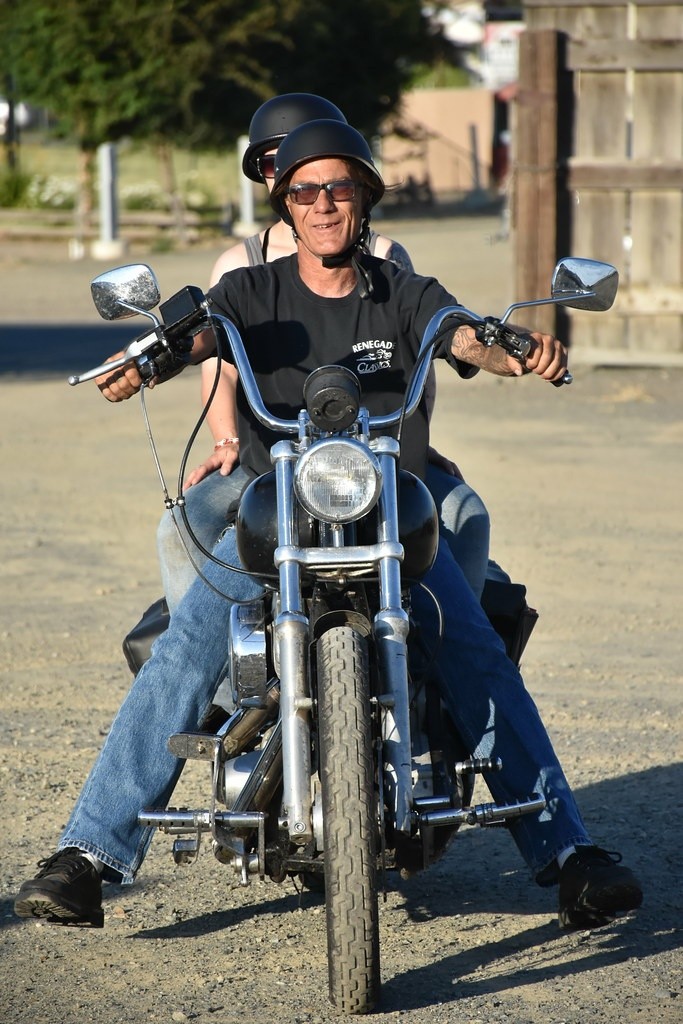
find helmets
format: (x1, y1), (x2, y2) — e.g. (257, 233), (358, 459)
(268, 119), (385, 226)
(241, 96), (350, 184)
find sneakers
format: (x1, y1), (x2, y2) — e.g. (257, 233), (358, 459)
(14, 848), (105, 928)
(558, 844), (643, 931)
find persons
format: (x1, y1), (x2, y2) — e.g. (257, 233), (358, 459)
(13, 119), (642, 929)
(160, 93), (490, 678)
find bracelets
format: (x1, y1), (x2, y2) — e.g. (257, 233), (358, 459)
(215, 437), (239, 451)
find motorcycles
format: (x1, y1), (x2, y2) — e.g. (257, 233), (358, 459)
(64, 257), (621, 1016)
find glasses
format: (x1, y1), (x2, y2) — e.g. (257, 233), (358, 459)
(280, 180), (369, 205)
(255, 154), (276, 178)
